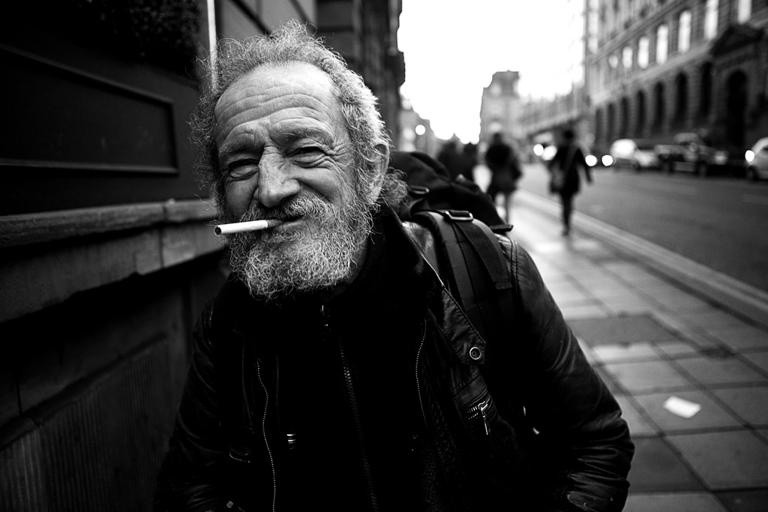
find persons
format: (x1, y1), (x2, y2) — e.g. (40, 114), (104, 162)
(546, 127), (596, 237)
(435, 142), (458, 170)
(448, 142), (477, 183)
(484, 131), (518, 225)
(159, 17), (639, 511)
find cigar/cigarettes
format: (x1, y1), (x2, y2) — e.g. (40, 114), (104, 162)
(213, 218), (284, 236)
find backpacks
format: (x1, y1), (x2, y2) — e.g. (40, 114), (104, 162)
(379, 149), (512, 243)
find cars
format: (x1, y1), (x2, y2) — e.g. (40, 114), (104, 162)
(609, 136), (661, 171)
(655, 128), (744, 178)
(743, 137), (768, 182)
